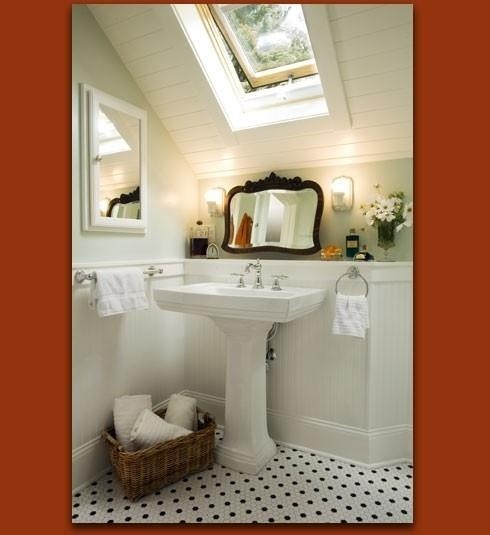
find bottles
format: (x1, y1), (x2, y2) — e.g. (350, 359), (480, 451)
(345, 227), (361, 257)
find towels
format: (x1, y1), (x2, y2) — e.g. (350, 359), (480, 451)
(164, 393), (201, 431)
(331, 292), (371, 339)
(89, 266), (151, 317)
(130, 413), (194, 449)
(112, 393), (153, 449)
(233, 212), (253, 247)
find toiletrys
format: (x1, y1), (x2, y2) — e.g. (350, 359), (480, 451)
(346, 227), (359, 261)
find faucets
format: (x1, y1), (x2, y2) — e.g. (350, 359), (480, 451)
(245, 257), (265, 289)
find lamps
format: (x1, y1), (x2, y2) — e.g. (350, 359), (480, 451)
(329, 176), (355, 214)
(204, 186), (226, 218)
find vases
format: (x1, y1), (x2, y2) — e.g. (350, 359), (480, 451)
(377, 221), (396, 262)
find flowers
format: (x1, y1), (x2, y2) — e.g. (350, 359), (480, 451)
(359, 184), (413, 234)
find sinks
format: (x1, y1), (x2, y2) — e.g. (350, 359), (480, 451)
(150, 281), (326, 337)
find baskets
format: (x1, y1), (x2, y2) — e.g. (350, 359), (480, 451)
(100, 397), (217, 503)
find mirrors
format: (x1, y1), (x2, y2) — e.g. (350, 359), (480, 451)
(220, 172), (324, 255)
(106, 185), (140, 219)
(86, 88), (148, 232)
